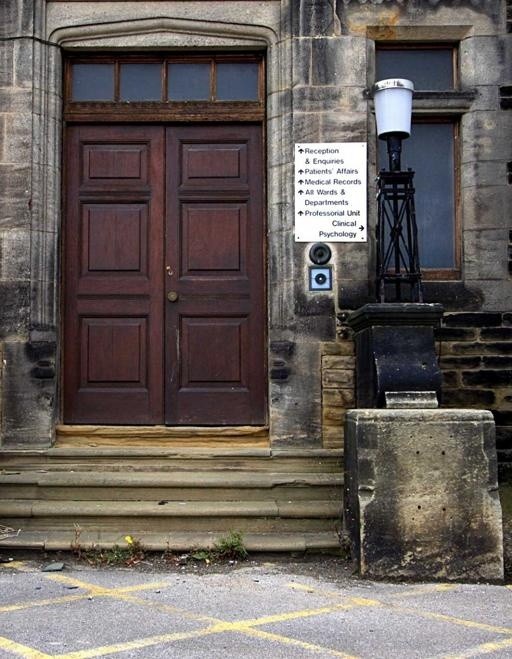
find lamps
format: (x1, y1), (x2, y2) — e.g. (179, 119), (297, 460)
(368, 76), (422, 304)
